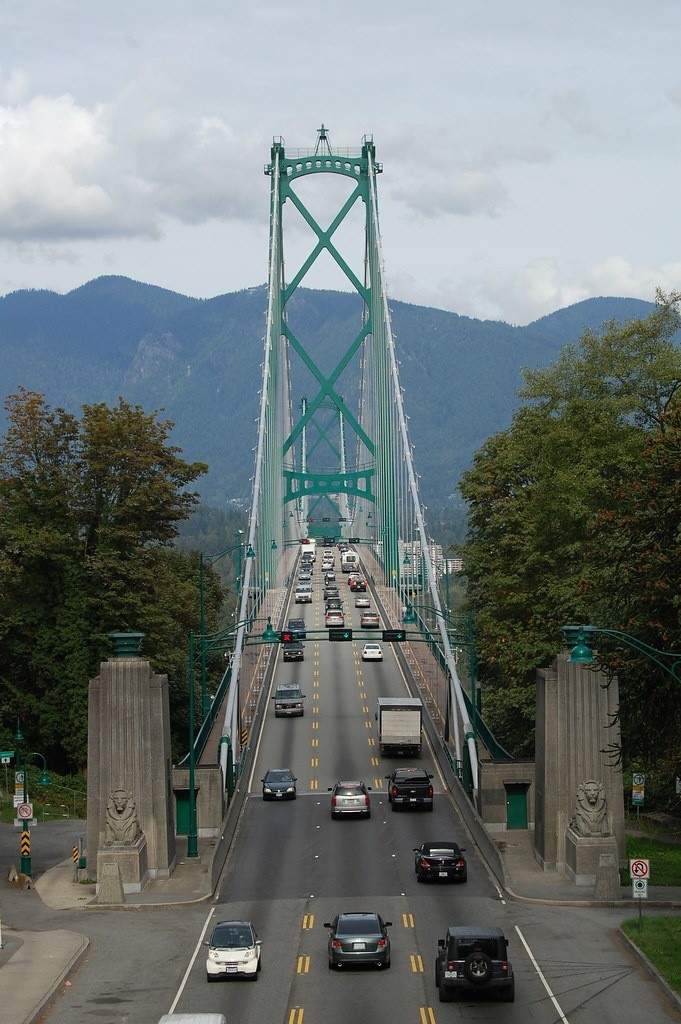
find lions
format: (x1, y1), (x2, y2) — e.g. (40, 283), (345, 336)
(570, 780), (613, 837)
(104, 789), (143, 844)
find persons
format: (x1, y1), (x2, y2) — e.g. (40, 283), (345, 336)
(239, 932), (250, 941)
(283, 774), (289, 780)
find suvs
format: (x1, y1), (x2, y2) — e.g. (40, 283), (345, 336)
(286, 618), (307, 639)
(434, 926), (515, 1003)
(384, 767), (434, 812)
(202, 920), (263, 982)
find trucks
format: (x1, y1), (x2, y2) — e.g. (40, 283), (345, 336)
(374, 697), (423, 759)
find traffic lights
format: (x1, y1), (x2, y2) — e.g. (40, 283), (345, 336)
(329, 629), (352, 641)
(382, 629), (406, 642)
(280, 631), (293, 642)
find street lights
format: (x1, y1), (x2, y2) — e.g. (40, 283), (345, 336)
(401, 603), (478, 739)
(18, 752), (51, 879)
(14, 717), (25, 772)
(402, 550), (452, 643)
(186, 616), (279, 859)
(198, 541), (256, 723)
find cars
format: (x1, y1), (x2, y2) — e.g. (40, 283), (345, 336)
(323, 609), (345, 628)
(323, 911), (394, 971)
(327, 780), (373, 820)
(360, 611), (380, 628)
(294, 537), (371, 615)
(361, 643), (383, 662)
(282, 641), (305, 662)
(413, 841), (468, 884)
(261, 768), (298, 801)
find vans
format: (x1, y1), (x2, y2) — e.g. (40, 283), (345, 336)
(270, 683), (306, 718)
